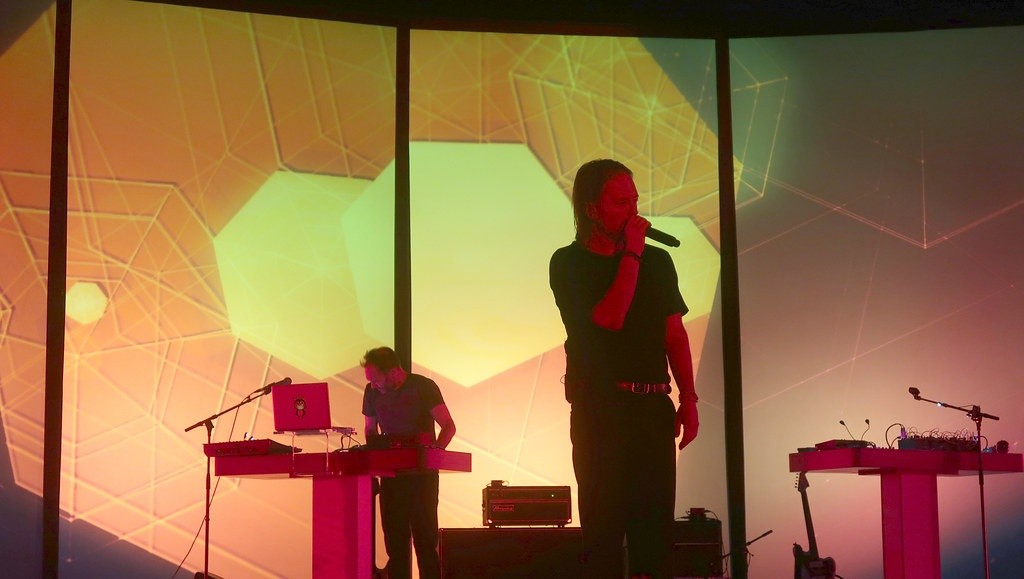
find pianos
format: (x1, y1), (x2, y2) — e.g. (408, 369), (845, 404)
(789, 452), (1024, 476)
(215, 449), (471, 477)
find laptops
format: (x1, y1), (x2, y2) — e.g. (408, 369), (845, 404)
(271, 382), (355, 430)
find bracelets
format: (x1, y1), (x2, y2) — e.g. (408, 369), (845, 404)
(622, 250), (640, 262)
(679, 391), (699, 403)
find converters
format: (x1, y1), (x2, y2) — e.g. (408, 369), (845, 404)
(689, 508), (706, 521)
(491, 480), (503, 486)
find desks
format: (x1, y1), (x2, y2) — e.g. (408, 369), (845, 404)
(788, 448), (1024, 579)
(215, 450), (473, 579)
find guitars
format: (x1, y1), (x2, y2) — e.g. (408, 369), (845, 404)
(793, 470), (836, 579)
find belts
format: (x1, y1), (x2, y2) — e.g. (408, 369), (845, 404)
(611, 378), (672, 395)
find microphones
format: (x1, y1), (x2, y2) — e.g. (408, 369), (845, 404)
(840, 420), (855, 441)
(254, 377), (292, 393)
(861, 419), (870, 440)
(645, 225), (680, 247)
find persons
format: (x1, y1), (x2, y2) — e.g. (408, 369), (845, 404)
(549, 159), (699, 579)
(362, 347), (456, 579)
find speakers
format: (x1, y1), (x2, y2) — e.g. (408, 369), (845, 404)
(438, 527), (585, 579)
(673, 520), (722, 579)
(483, 486), (572, 526)
